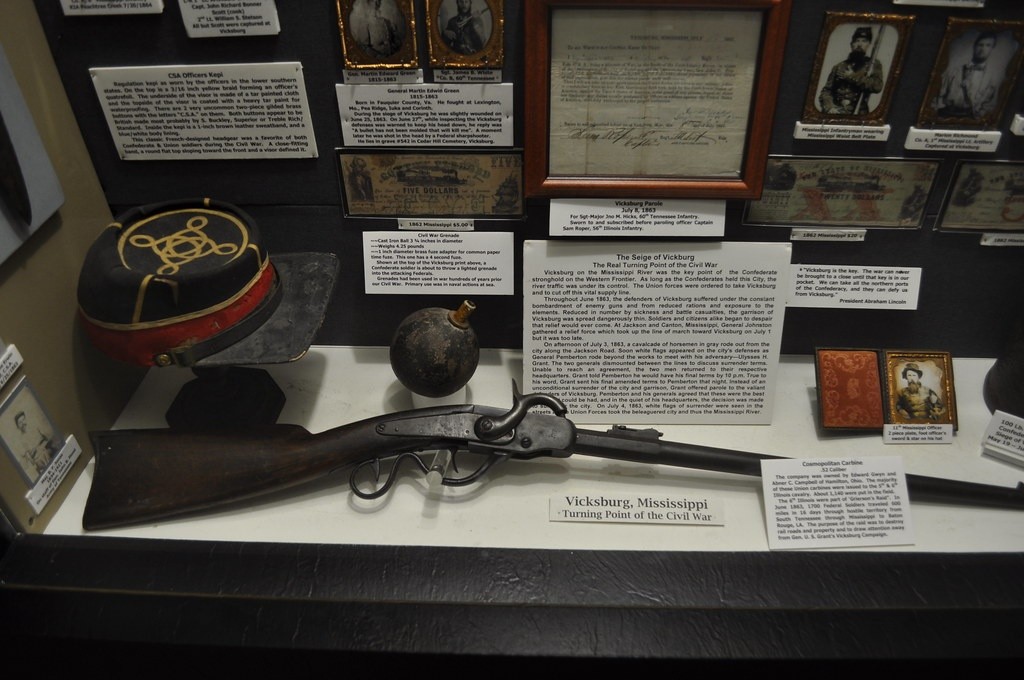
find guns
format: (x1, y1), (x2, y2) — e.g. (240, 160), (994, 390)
(81, 372), (1024, 533)
(850, 21), (889, 118)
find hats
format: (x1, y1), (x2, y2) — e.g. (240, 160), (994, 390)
(77, 199), (283, 368)
(902, 362), (923, 380)
(852, 27), (873, 44)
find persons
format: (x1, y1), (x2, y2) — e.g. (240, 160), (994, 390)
(921, 27), (996, 121)
(348, 158), (374, 202)
(357, 1), (396, 56)
(15, 410), (58, 476)
(894, 362), (943, 418)
(445, 0), (487, 55)
(818, 23), (885, 117)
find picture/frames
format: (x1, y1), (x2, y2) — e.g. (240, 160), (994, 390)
(336, 0), (419, 71)
(915, 15), (1024, 131)
(813, 346), (887, 436)
(929, 156), (1024, 235)
(425, 0), (504, 69)
(333, 147), (528, 223)
(522, 0), (792, 201)
(882, 347), (959, 432)
(800, 10), (917, 127)
(740, 153), (945, 231)
